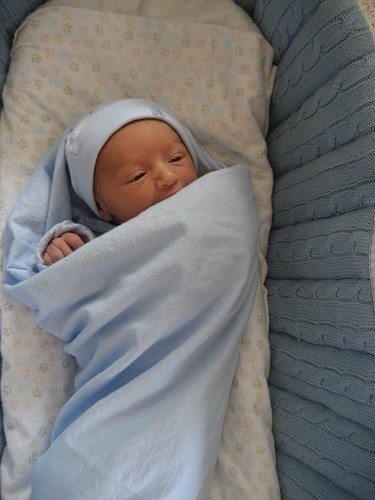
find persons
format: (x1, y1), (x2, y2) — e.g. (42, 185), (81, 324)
(6, 101), (258, 499)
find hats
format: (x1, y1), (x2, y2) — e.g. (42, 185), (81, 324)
(65, 98), (198, 220)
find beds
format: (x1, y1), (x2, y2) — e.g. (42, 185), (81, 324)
(1, 0), (375, 500)
